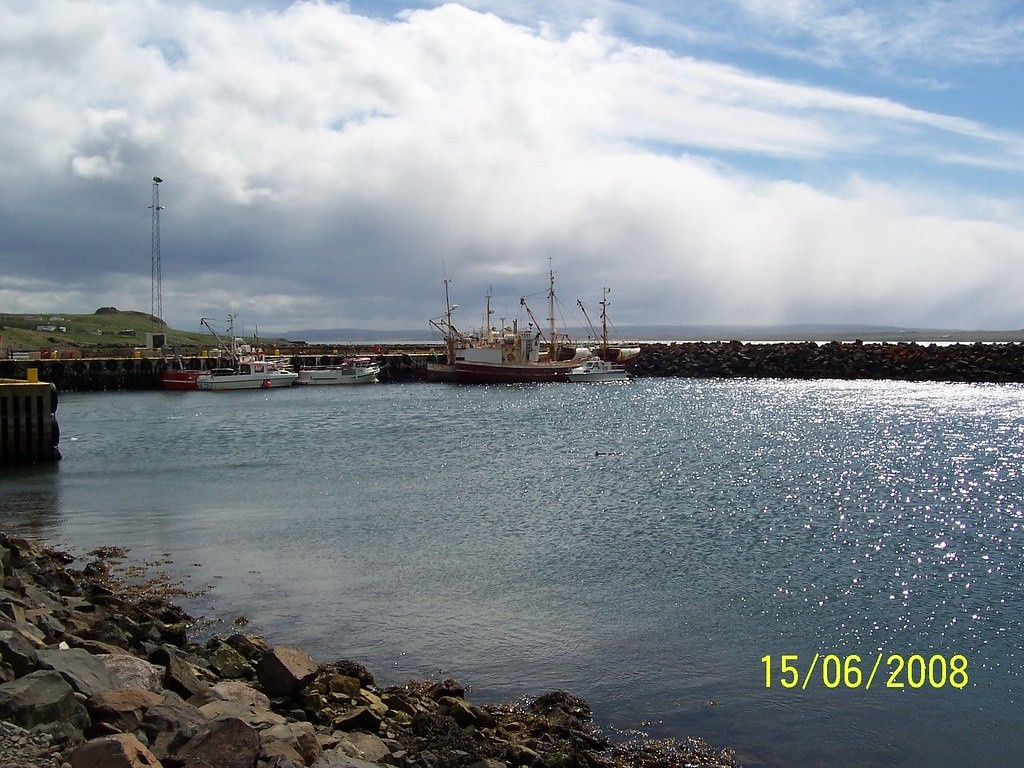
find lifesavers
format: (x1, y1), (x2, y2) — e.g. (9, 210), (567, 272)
(1, 359), (219, 377)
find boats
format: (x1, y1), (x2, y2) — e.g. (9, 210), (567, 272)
(295, 357), (381, 384)
(565, 356), (629, 381)
(162, 313), (294, 390)
(196, 361), (299, 389)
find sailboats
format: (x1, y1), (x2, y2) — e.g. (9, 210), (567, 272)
(426, 256), (641, 382)
